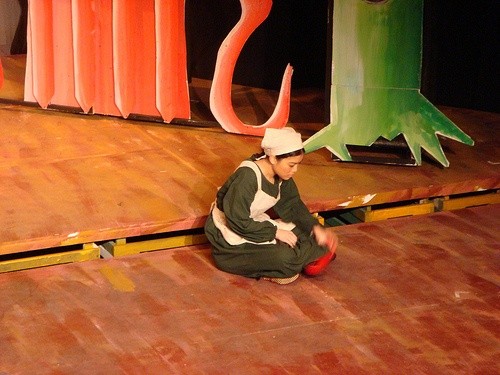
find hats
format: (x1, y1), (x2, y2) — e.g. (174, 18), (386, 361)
(261, 127), (304, 156)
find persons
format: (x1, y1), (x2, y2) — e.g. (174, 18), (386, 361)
(205, 126), (338, 284)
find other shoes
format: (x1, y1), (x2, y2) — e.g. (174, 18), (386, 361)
(305, 249), (332, 276)
